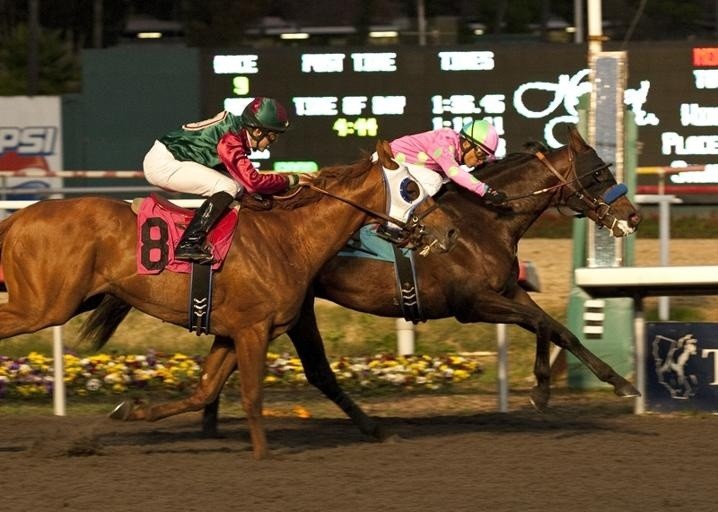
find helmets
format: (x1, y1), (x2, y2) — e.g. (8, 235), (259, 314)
(241, 97), (289, 131)
(460, 119), (498, 155)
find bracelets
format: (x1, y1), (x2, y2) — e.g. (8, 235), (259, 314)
(287, 174), (299, 186)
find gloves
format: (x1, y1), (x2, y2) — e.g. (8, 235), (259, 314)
(290, 173), (320, 184)
(483, 187), (507, 204)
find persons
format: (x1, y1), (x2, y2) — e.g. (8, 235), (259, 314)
(142, 94), (313, 263)
(369, 119), (507, 249)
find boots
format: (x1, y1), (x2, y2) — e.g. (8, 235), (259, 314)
(174, 191), (233, 260)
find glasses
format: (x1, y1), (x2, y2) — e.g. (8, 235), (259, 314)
(247, 109), (278, 143)
(471, 121), (485, 157)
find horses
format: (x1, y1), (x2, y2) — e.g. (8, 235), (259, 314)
(73, 122), (643, 438)
(0, 139), (462, 462)
